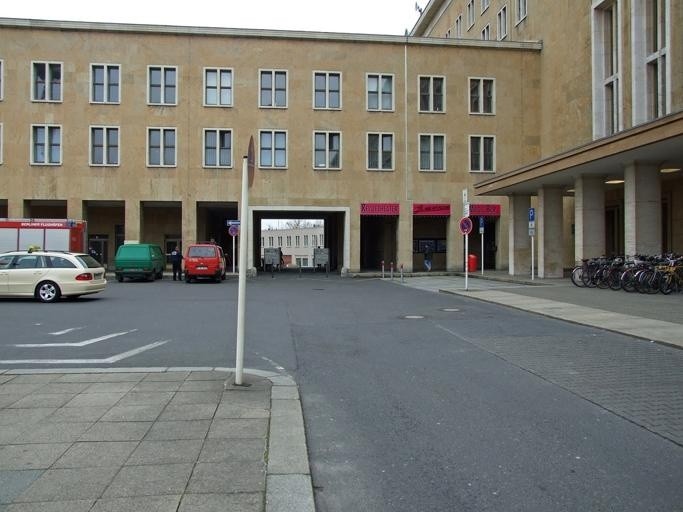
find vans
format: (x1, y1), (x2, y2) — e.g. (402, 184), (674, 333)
(114, 244), (164, 282)
(184, 245), (226, 282)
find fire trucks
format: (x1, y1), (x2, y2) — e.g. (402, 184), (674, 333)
(0, 218), (87, 253)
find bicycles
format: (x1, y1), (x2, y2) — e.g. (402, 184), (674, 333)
(571, 253), (683, 295)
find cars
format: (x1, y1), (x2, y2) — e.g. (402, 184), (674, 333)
(0, 247), (107, 302)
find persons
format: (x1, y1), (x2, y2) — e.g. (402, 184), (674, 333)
(423, 243), (433, 272)
(316, 246), (321, 270)
(276, 247), (285, 272)
(169, 246), (185, 281)
(88, 246), (96, 259)
